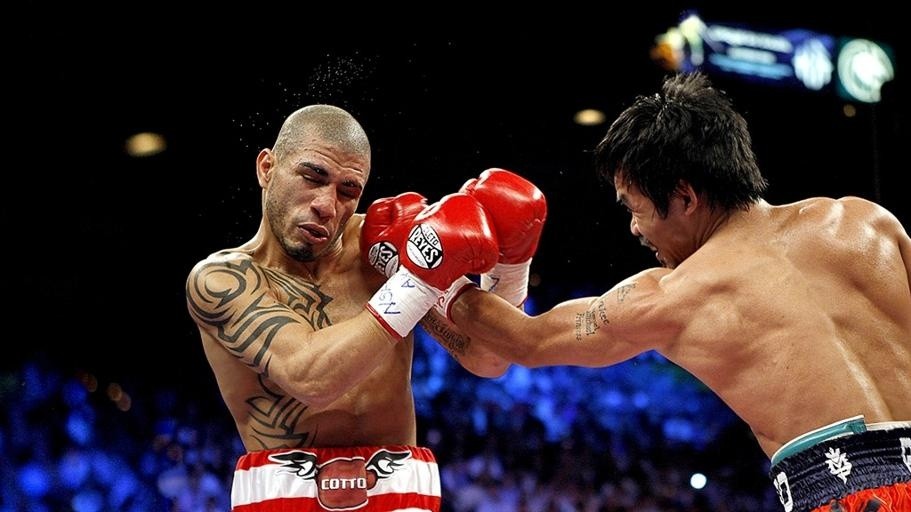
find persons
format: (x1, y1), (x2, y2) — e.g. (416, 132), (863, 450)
(185, 104), (548, 512)
(360, 71), (911, 512)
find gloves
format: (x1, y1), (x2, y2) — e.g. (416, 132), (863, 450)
(359, 167), (547, 293)
